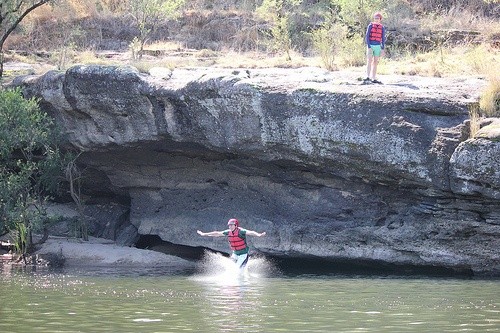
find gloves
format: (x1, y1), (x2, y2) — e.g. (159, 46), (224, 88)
(368, 44), (371, 48)
(381, 46), (384, 49)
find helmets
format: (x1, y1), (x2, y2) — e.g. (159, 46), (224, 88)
(228, 218), (239, 225)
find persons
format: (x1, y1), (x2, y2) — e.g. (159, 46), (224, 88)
(363, 13), (386, 82)
(196, 218), (267, 267)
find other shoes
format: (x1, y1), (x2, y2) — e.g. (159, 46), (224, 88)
(364, 78), (381, 83)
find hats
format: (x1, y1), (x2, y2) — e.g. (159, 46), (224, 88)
(373, 14), (382, 19)
